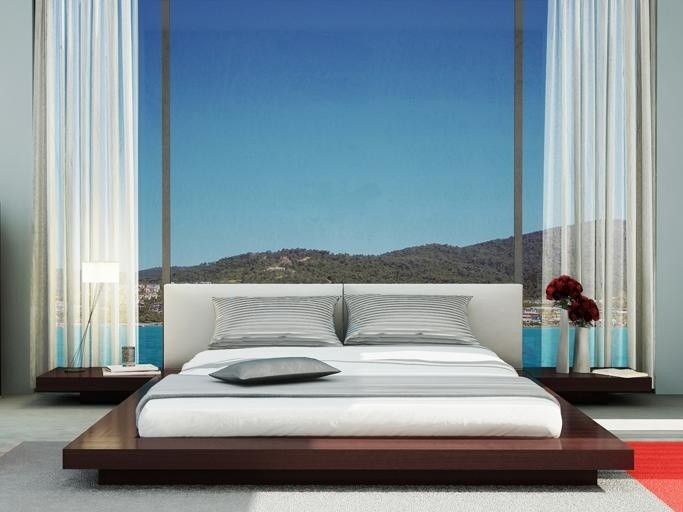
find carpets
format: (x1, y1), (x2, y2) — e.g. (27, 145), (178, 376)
(0, 440), (683, 512)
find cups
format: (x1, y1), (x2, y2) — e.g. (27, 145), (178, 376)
(121, 347), (136, 368)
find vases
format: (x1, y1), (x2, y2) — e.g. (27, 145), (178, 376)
(573, 325), (591, 374)
(556, 309), (570, 373)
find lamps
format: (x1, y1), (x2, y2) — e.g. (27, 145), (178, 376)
(63, 262), (120, 373)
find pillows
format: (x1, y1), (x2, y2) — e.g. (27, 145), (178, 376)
(207, 295), (343, 350)
(208, 356), (342, 385)
(344, 294), (481, 345)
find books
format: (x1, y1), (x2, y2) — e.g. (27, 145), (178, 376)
(102, 363), (162, 377)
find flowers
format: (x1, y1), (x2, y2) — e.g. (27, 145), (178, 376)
(568, 295), (600, 327)
(546, 275), (584, 310)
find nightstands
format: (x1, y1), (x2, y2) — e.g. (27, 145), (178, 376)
(33, 366), (161, 405)
(523, 366), (652, 406)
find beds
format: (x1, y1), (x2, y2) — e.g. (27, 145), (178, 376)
(63, 284), (634, 487)
